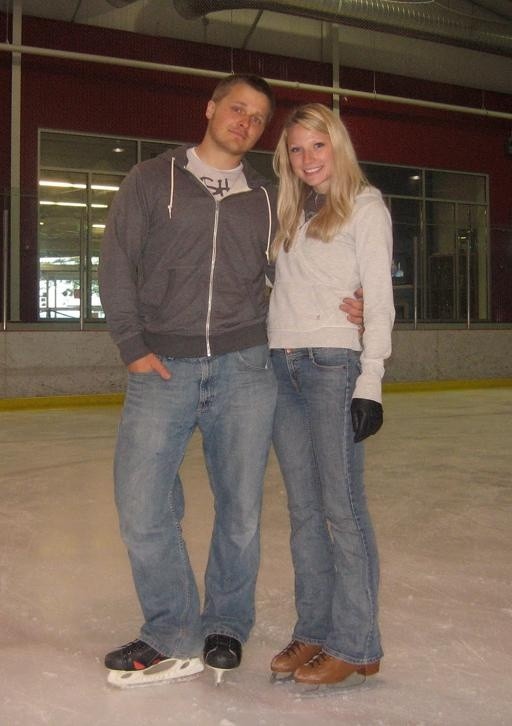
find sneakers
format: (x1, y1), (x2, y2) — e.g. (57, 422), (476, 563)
(105, 638), (170, 671)
(204, 634), (241, 669)
(271, 639), (379, 684)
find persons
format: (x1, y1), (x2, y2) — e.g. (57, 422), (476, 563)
(261, 100), (396, 687)
(96, 73), (366, 671)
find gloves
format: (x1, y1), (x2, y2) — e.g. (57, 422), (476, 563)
(350, 398), (382, 443)
